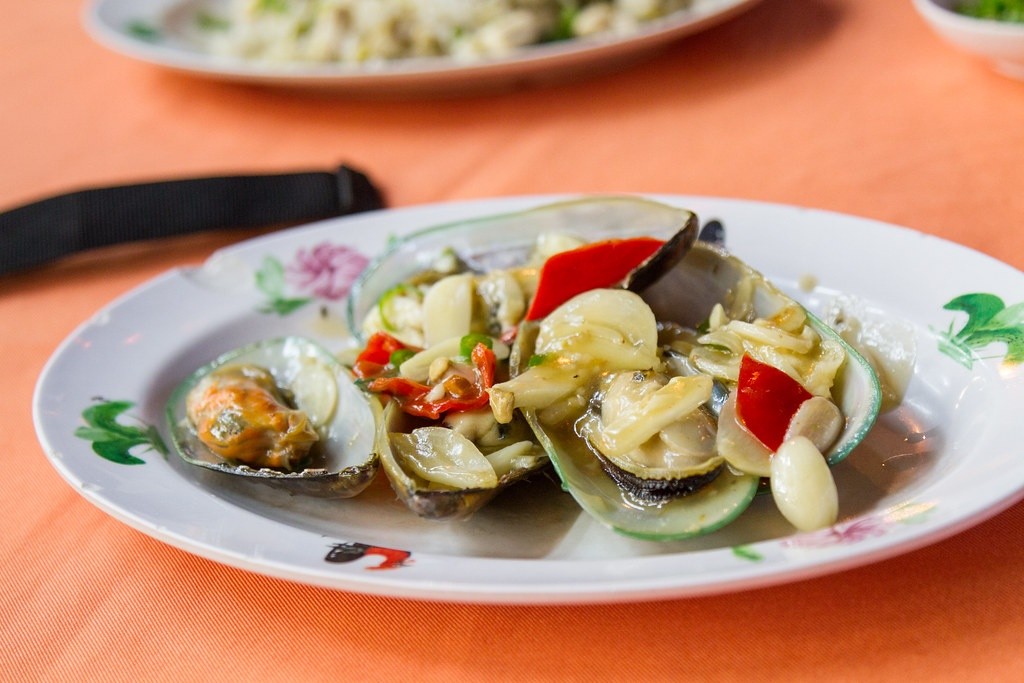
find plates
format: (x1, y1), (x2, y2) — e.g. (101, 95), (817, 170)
(80, 0), (764, 103)
(26, 188), (1024, 614)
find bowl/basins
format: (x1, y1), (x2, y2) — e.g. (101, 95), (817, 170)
(913, 2), (1022, 86)
